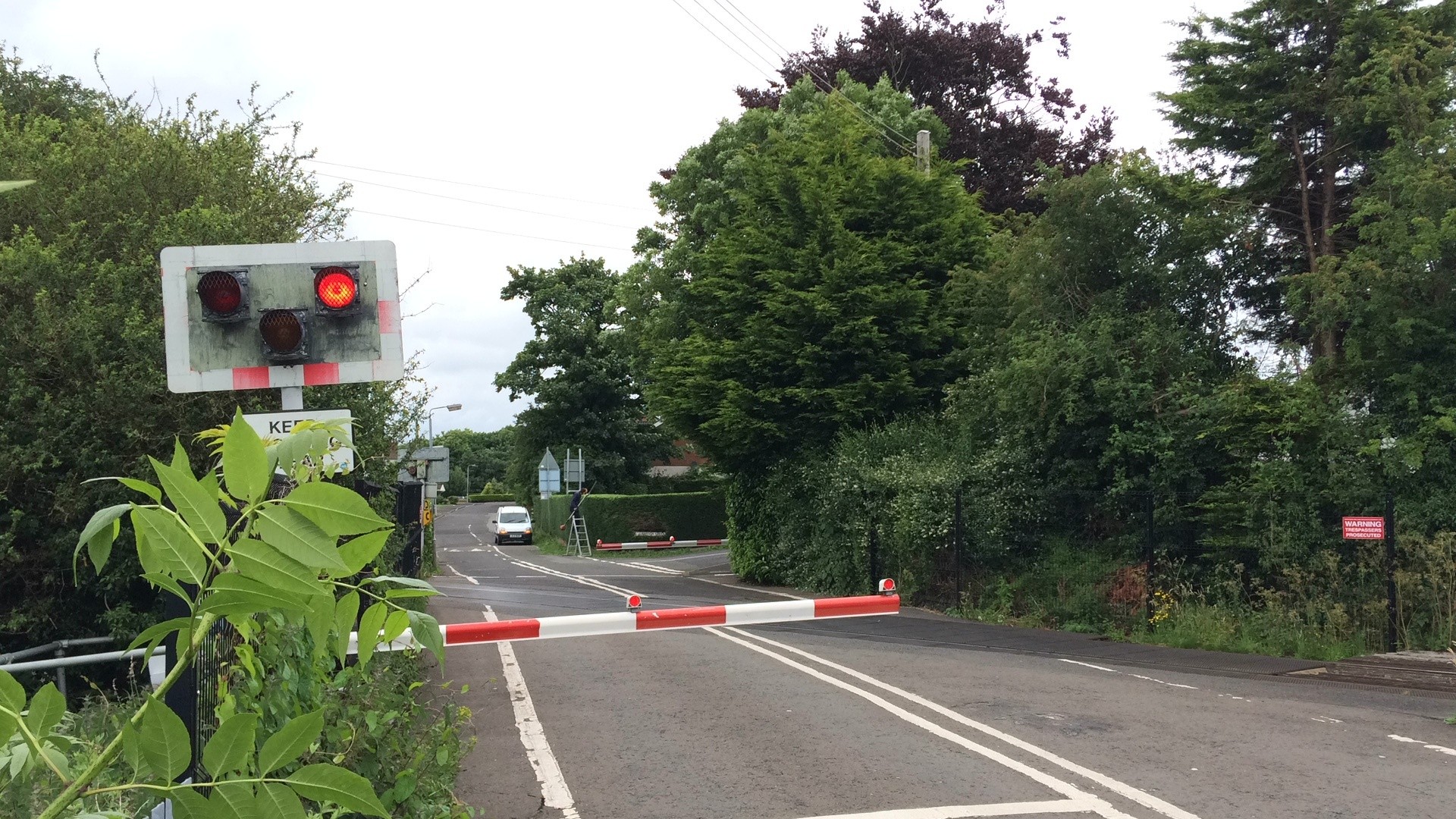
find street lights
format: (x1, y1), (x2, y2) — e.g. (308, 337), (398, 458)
(428, 404), (462, 447)
(467, 464), (475, 502)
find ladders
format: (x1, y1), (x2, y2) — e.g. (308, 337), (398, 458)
(566, 515), (592, 557)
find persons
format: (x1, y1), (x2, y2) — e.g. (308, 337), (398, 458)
(569, 487), (586, 535)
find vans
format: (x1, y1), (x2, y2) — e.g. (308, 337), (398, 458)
(492, 506), (536, 545)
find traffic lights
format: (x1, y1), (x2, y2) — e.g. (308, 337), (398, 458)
(310, 264), (363, 318)
(195, 267), (252, 324)
(258, 307), (310, 362)
